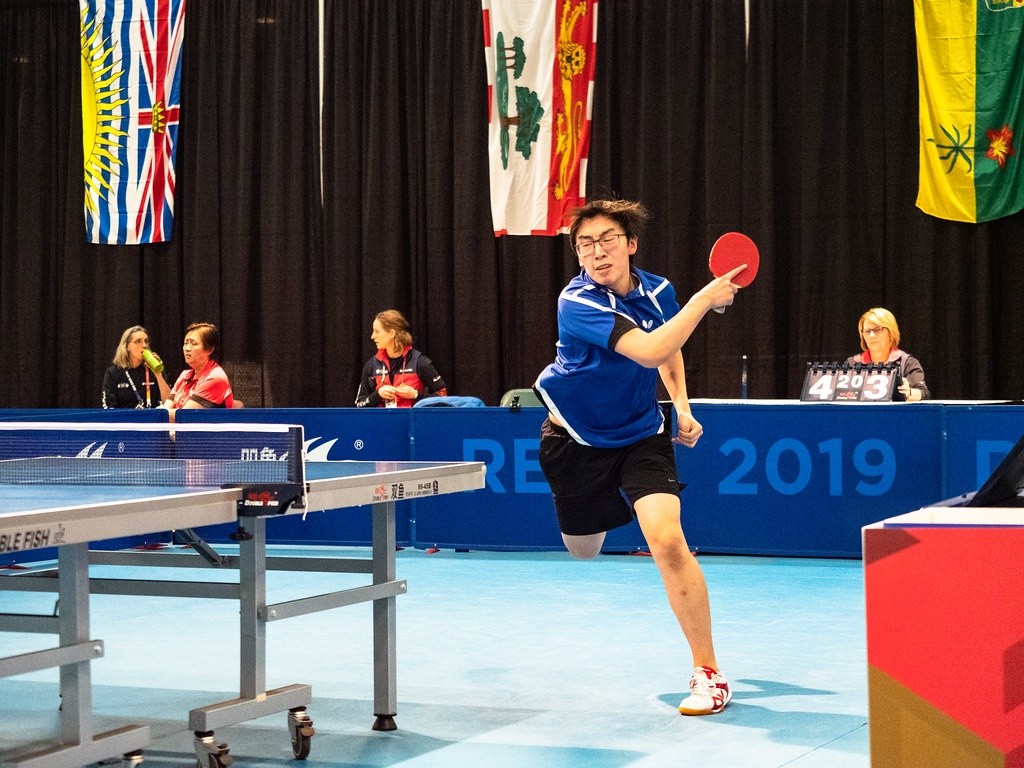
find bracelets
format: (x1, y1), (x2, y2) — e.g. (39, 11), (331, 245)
(906, 389), (911, 399)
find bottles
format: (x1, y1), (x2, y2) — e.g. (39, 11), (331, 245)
(142, 350), (165, 373)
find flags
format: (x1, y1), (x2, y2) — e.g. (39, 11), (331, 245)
(480, 1), (599, 238)
(78, 0), (187, 251)
(913, 0), (1023, 224)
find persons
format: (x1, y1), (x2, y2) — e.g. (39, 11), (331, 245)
(102, 325), (171, 408)
(846, 308), (933, 403)
(167, 323), (233, 411)
(531, 201), (749, 715)
(355, 308), (449, 408)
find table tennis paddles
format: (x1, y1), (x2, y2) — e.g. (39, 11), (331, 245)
(708, 232), (758, 314)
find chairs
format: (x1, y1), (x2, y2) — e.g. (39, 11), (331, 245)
(225, 363), (290, 407)
(500, 389), (543, 406)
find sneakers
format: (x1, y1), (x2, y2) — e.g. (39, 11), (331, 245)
(678, 665), (732, 715)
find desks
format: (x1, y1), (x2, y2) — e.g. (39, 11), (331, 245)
(0, 407), (1024, 559)
(0, 423), (486, 768)
(862, 492), (1024, 768)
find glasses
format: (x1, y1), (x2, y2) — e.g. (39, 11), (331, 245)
(861, 326), (888, 335)
(127, 338), (150, 346)
(574, 233), (627, 257)
